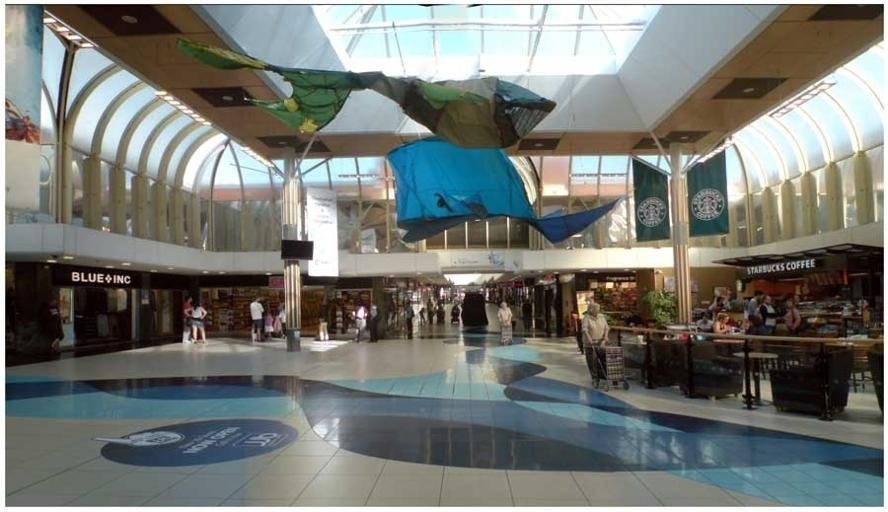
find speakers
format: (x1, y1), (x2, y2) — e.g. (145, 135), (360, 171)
(281, 239), (313, 259)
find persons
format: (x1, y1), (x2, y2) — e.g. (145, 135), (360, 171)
(497, 301), (512, 328)
(319, 304), (329, 341)
(581, 303), (610, 381)
(184, 301), (207, 344)
(571, 311), (584, 355)
(182, 296), (193, 343)
(697, 289), (801, 335)
(46, 298), (64, 353)
(249, 296), (286, 342)
(355, 299), (461, 342)
(522, 299), (533, 332)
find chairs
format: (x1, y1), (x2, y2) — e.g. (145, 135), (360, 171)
(609, 325), (883, 422)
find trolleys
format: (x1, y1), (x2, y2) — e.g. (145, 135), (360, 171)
(499, 323), (513, 345)
(586, 341), (630, 392)
